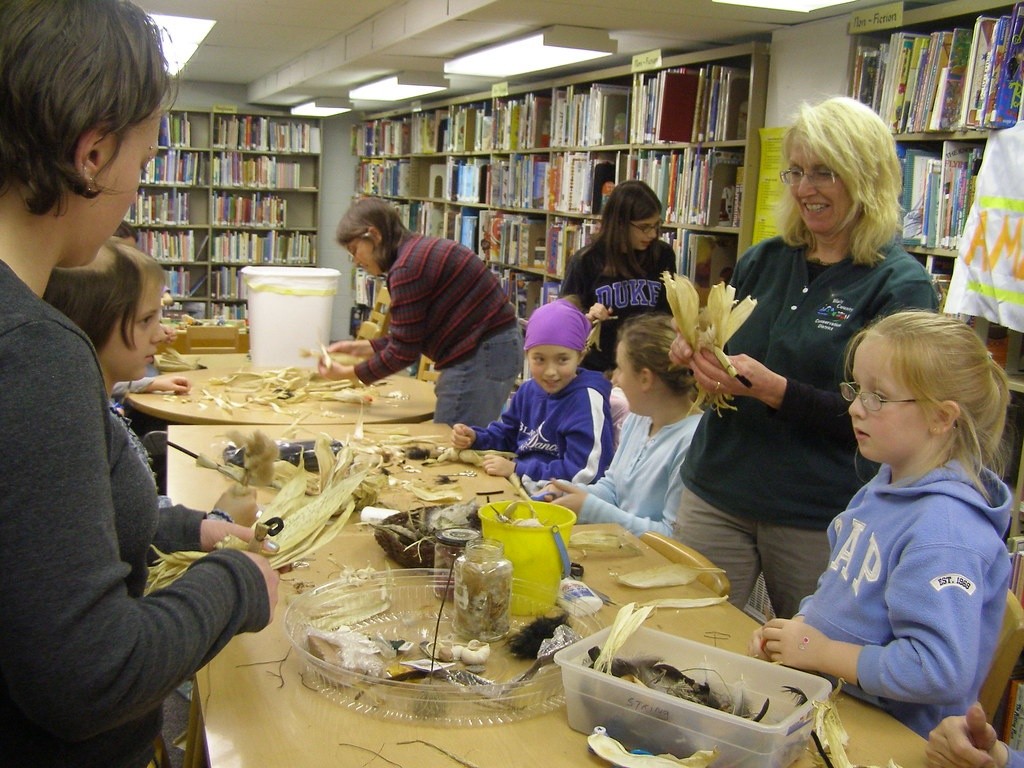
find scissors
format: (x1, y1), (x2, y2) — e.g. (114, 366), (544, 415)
(438, 470), (477, 477)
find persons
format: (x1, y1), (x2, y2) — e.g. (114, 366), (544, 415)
(674, 96), (942, 620)
(0, 0), (279, 768)
(558, 180), (676, 373)
(42, 221), (261, 528)
(924, 704), (1024, 768)
(450, 300), (705, 539)
(753, 308), (1012, 742)
(318, 196), (525, 427)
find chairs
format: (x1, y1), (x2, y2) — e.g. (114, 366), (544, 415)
(418, 352), (441, 384)
(638, 530), (730, 597)
(978, 588), (1024, 725)
(354, 279), (393, 339)
(187, 325), (240, 354)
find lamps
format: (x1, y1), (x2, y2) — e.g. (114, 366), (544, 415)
(444, 25), (618, 77)
(349, 71), (450, 101)
(290, 98), (352, 117)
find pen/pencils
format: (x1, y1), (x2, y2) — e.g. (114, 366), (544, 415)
(167, 440), (198, 459)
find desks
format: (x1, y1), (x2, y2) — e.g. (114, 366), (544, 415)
(128, 324), (933, 768)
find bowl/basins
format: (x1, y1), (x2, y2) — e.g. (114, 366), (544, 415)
(283, 568), (607, 725)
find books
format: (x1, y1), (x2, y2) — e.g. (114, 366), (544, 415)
(847, 0), (1024, 376)
(211, 114), (320, 325)
(123, 112), (208, 322)
(350, 63), (749, 336)
(993, 402), (1024, 753)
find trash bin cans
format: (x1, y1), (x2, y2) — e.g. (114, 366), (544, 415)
(237, 265), (341, 375)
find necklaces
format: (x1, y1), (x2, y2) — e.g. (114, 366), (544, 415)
(109, 407), (132, 426)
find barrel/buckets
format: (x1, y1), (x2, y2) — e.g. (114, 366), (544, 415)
(478, 500), (577, 615)
(240, 266), (342, 367)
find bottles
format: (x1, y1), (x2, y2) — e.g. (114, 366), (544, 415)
(558, 576), (603, 615)
(452, 539), (513, 643)
(434, 526), (483, 601)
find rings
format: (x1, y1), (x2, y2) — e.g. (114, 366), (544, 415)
(715, 381), (720, 391)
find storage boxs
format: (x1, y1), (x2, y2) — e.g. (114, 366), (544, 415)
(554, 624), (832, 768)
(242, 265), (342, 369)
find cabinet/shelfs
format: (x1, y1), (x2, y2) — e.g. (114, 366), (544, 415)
(349, 42), (771, 400)
(122, 107), (323, 319)
(841, 0), (1024, 753)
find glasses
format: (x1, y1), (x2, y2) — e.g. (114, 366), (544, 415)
(780, 167), (840, 187)
(629, 218), (664, 233)
(839, 381), (917, 412)
